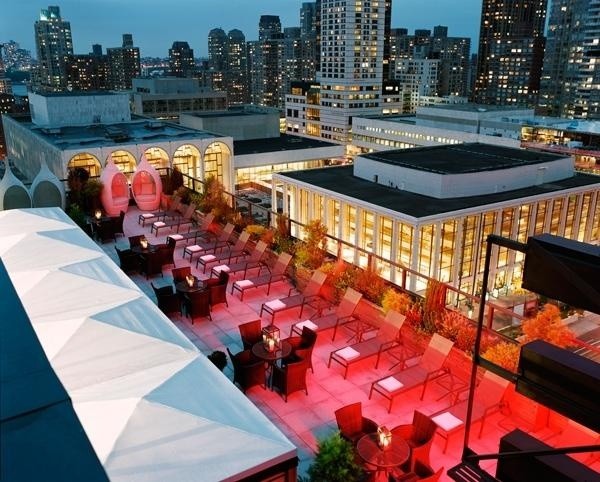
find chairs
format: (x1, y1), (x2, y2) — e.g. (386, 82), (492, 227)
(365, 331), (458, 415)
(267, 354), (311, 403)
(385, 457), (447, 482)
(325, 309), (409, 379)
(225, 346), (267, 395)
(236, 316), (265, 351)
(290, 286), (362, 344)
(332, 400), (380, 444)
(390, 408), (440, 473)
(86, 147), (297, 323)
(283, 327), (319, 376)
(257, 270), (329, 324)
(426, 369), (515, 456)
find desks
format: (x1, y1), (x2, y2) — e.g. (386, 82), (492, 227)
(250, 339), (291, 362)
(354, 430), (412, 482)
(261, 324), (283, 342)
